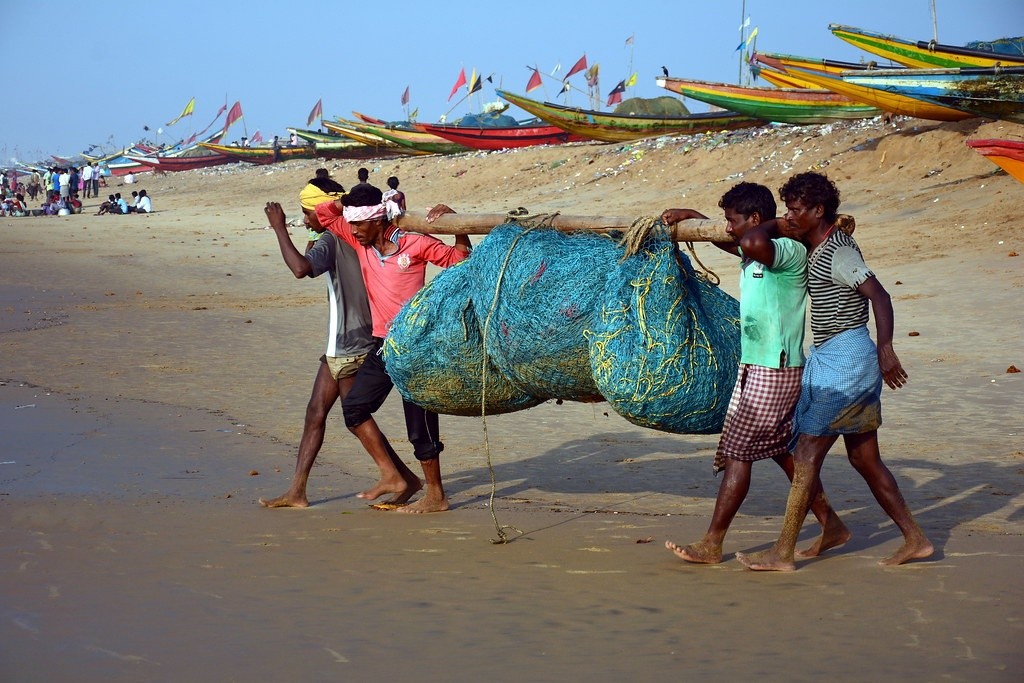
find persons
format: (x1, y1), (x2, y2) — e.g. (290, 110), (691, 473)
(0, 161), (151, 217)
(258, 167), (474, 513)
(660, 180), (851, 564)
(232, 134), (297, 164)
(736, 172), (935, 572)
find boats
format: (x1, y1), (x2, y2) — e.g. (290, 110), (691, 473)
(14, 124), (238, 176)
(495, 88), (767, 144)
(965, 138), (1024, 185)
(286, 111), (472, 156)
(415, 123), (592, 150)
(655, 23), (1024, 123)
(196, 142), (313, 165)
